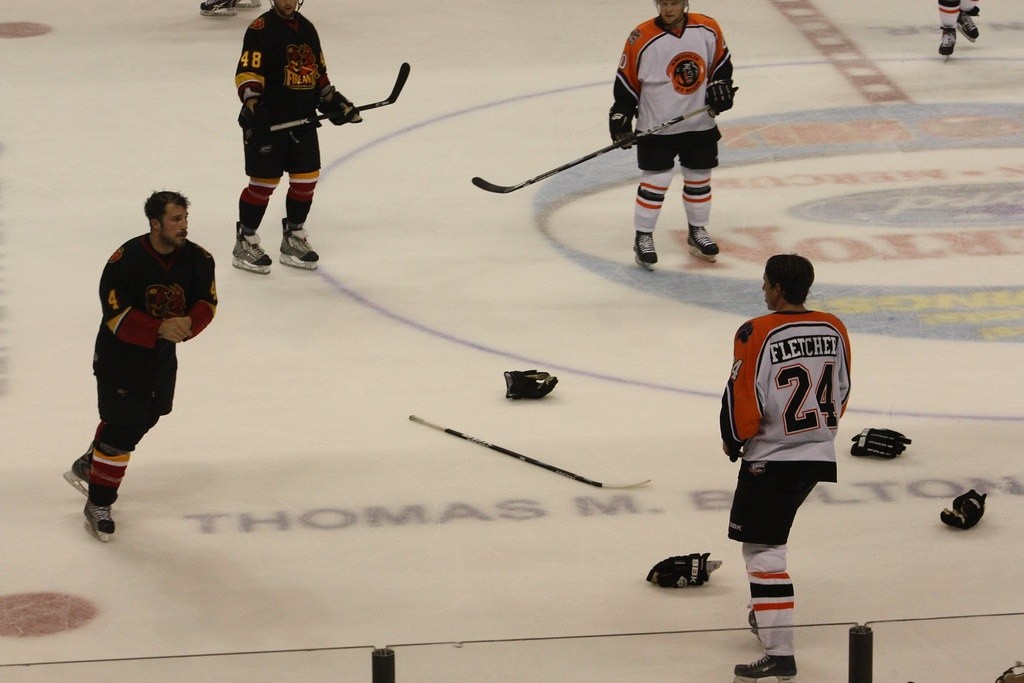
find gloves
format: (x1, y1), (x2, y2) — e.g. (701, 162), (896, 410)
(851, 428), (912, 459)
(238, 103), (272, 153)
(504, 370), (558, 400)
(609, 104), (637, 150)
(646, 553), (722, 589)
(941, 490), (987, 529)
(705, 83), (734, 118)
(318, 91), (362, 125)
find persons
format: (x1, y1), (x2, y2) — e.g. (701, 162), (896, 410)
(232, 0), (363, 266)
(200, 0), (235, 10)
(939, 1), (981, 55)
(608, 0), (736, 265)
(721, 254), (850, 678)
(72, 191), (218, 536)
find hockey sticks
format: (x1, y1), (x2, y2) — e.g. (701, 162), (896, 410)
(270, 61), (411, 131)
(408, 414), (654, 490)
(470, 86), (739, 195)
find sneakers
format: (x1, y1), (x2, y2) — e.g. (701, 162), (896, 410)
(688, 224), (719, 262)
(82, 497), (115, 542)
(733, 654), (797, 683)
(749, 609), (761, 641)
(937, 26), (957, 62)
(235, 0), (262, 8)
(279, 218), (319, 269)
(64, 440), (94, 496)
(634, 231), (658, 270)
(232, 222), (272, 273)
(201, 0), (240, 16)
(958, 10), (978, 42)
